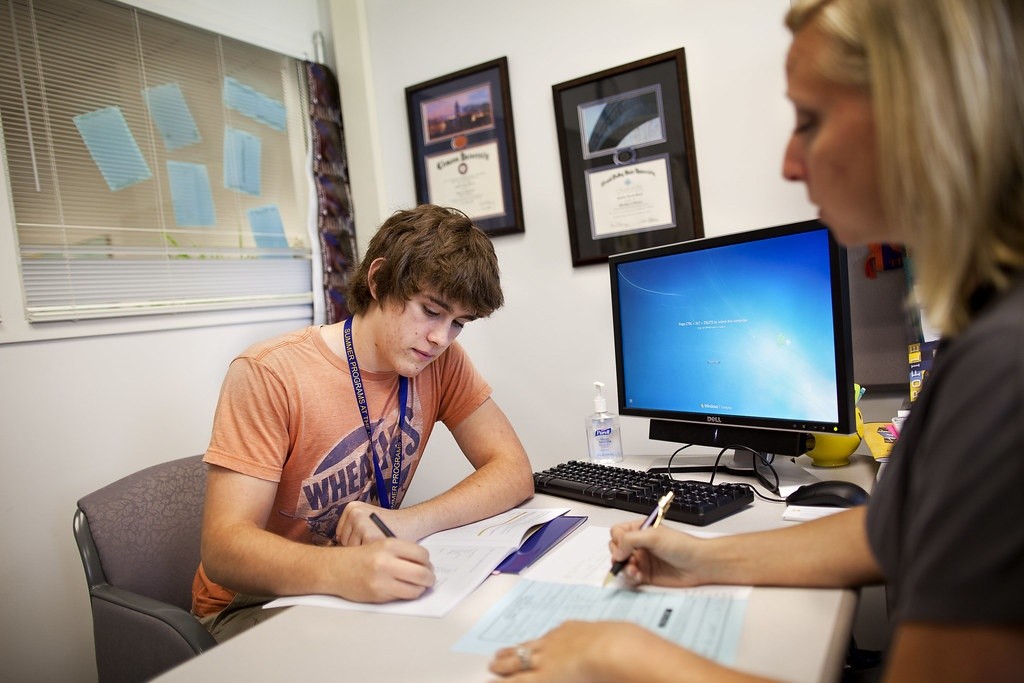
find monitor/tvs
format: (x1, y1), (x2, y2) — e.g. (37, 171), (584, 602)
(605, 218), (857, 500)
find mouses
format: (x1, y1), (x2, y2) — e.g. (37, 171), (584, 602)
(785, 481), (871, 509)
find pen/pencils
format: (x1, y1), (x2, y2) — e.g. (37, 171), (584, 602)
(370, 513), (396, 537)
(599, 489), (674, 590)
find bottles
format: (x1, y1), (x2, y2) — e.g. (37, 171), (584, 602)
(805, 408), (865, 468)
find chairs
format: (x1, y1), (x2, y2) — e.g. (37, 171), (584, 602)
(72, 454), (216, 683)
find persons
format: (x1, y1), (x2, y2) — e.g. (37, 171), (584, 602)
(190, 205), (534, 644)
(487, 0), (1023, 683)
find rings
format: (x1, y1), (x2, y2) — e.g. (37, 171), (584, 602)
(516, 647), (532, 671)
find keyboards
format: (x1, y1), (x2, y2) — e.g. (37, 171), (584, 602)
(534, 460), (753, 528)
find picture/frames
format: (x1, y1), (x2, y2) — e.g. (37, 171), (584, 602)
(551, 46), (707, 269)
(405, 55), (527, 241)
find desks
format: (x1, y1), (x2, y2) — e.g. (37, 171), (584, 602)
(141, 454), (877, 683)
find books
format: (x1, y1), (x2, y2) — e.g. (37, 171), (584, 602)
(864, 255), (942, 463)
(450, 523), (751, 670)
(262, 508), (589, 619)
